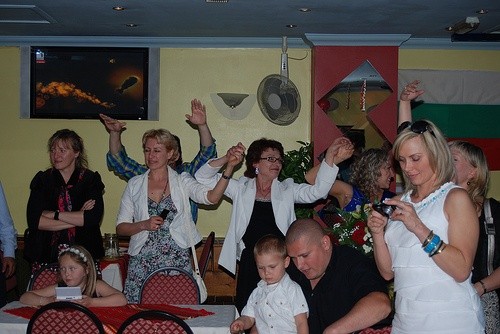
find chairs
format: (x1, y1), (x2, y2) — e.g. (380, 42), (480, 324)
(23, 227), (215, 334)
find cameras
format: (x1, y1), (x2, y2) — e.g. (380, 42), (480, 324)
(372, 203), (397, 217)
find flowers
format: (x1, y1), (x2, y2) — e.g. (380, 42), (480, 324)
(327, 204), (374, 254)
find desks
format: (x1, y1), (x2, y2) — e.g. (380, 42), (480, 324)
(0, 300), (244, 334)
(98, 247), (129, 293)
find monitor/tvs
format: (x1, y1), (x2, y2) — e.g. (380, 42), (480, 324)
(18, 45), (159, 121)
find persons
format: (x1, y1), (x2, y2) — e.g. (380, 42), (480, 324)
(367, 120), (485, 334)
(304, 141), (398, 218)
(99, 98), (218, 224)
(0, 181), (17, 278)
(194, 137), (351, 317)
(249, 218), (391, 334)
(27, 128), (106, 260)
(116, 129), (246, 303)
(229, 234), (310, 334)
(397, 80), (500, 334)
(20, 244), (128, 307)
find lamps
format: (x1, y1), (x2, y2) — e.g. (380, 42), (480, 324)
(217, 93), (249, 108)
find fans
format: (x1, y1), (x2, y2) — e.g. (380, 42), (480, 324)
(257, 36), (308, 126)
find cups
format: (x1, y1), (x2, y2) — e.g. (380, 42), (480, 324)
(105, 233), (120, 259)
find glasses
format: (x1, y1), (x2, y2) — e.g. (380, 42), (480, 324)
(397, 121), (436, 139)
(260, 157), (285, 164)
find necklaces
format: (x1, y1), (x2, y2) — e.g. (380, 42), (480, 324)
(258, 187), (270, 199)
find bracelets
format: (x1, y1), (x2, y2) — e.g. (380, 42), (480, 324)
(54, 209), (60, 220)
(222, 171), (232, 179)
(40, 296), (43, 304)
(478, 281), (486, 294)
(421, 230), (447, 257)
